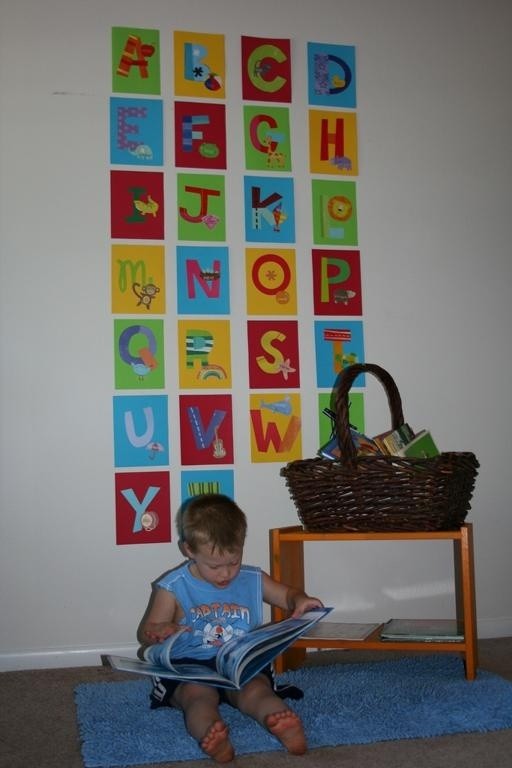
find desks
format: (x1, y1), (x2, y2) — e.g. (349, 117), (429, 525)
(269, 520), (485, 681)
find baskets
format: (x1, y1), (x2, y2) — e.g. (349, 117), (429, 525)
(280, 363), (480, 532)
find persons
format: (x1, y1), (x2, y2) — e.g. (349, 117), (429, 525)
(135, 493), (327, 765)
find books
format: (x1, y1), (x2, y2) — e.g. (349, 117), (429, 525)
(295, 617), (466, 644)
(98, 607), (335, 692)
(315, 423), (441, 459)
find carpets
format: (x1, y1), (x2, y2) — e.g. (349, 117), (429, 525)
(76, 651), (512, 768)
